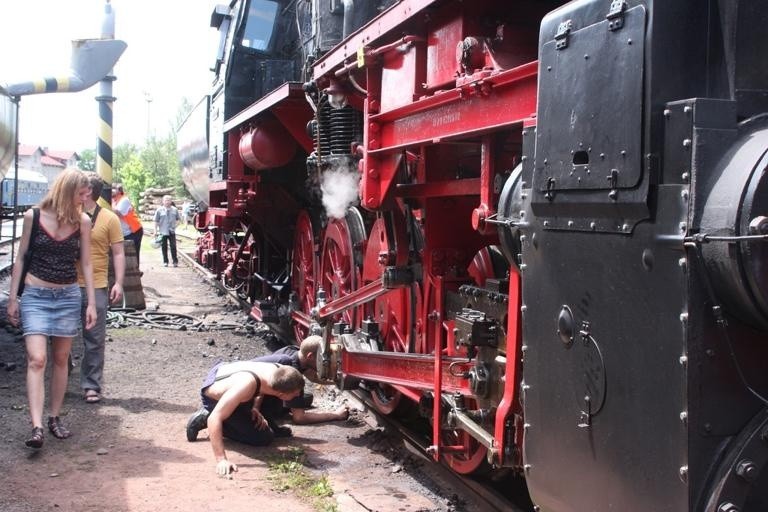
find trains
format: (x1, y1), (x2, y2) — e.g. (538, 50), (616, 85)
(0, 167), (49, 218)
(177, 0), (768, 512)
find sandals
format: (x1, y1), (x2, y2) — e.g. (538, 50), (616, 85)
(25, 426), (44, 449)
(48, 416), (73, 439)
(85, 388), (101, 404)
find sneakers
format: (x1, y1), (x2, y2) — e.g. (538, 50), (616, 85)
(164, 263), (177, 267)
(187, 402), (210, 442)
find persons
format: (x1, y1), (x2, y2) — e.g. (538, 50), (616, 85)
(154, 195), (180, 267)
(6, 165), (143, 449)
(186, 334), (350, 475)
(182, 198), (190, 230)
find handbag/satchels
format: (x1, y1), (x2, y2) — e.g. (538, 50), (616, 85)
(17, 250), (32, 297)
